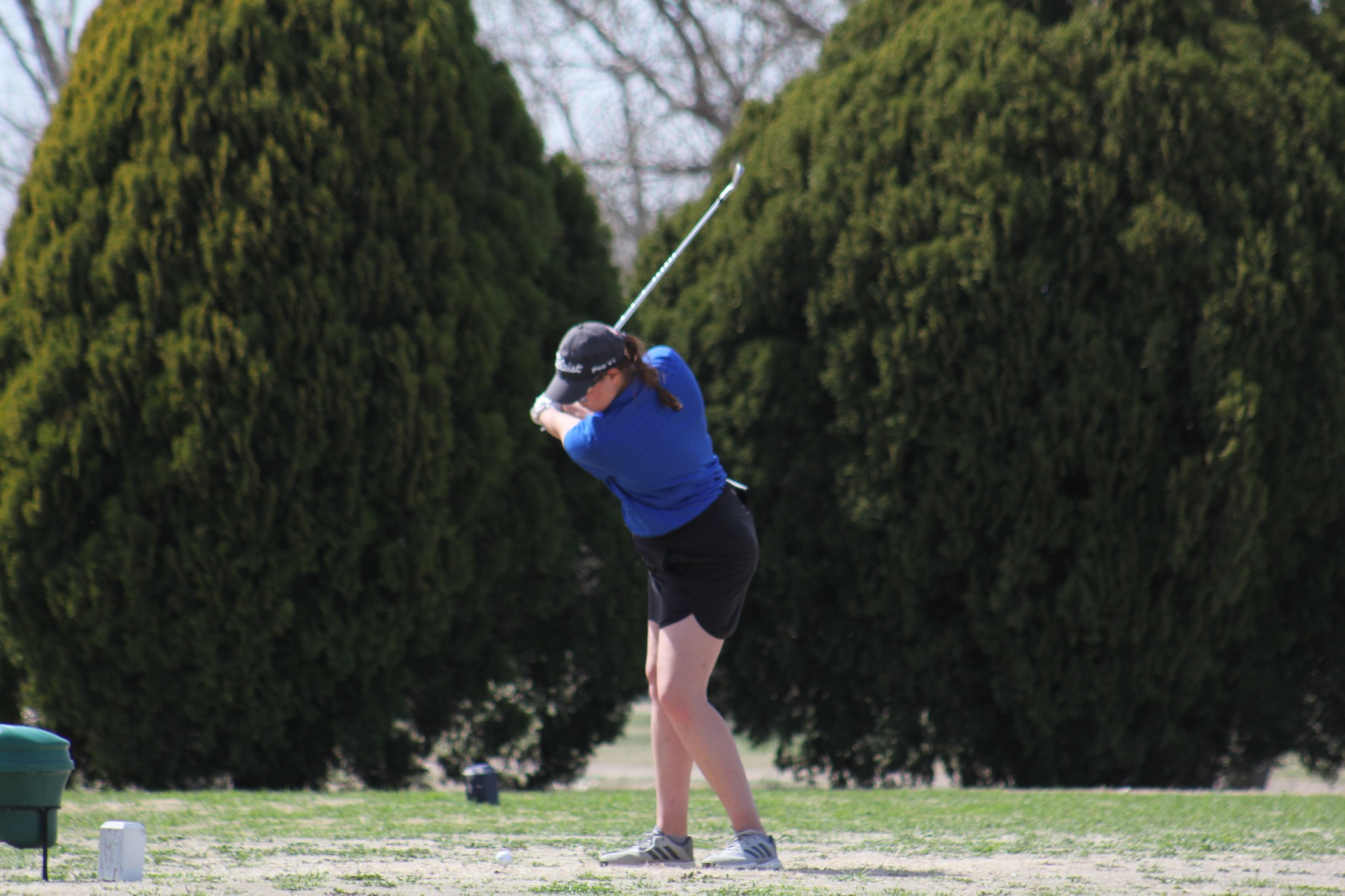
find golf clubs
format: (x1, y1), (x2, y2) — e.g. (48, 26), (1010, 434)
(538, 160), (745, 433)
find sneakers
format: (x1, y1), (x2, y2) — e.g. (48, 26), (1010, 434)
(598, 826), (696, 868)
(701, 829), (782, 870)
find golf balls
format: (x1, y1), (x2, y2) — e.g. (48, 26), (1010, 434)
(496, 850), (511, 867)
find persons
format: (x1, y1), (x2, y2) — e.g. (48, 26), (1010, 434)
(529, 322), (781, 870)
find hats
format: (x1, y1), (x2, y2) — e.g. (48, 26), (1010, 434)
(543, 322), (624, 405)
